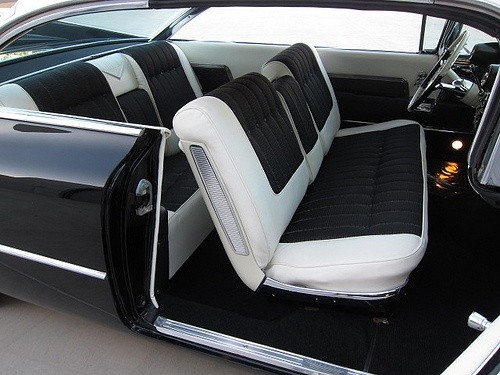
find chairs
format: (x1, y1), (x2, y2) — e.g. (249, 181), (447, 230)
(0, 60), (215, 280)
(171, 71), (430, 315)
(262, 41), (429, 176)
(119, 39), (205, 129)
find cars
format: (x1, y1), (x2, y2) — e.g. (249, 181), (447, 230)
(0, 0), (500, 375)
